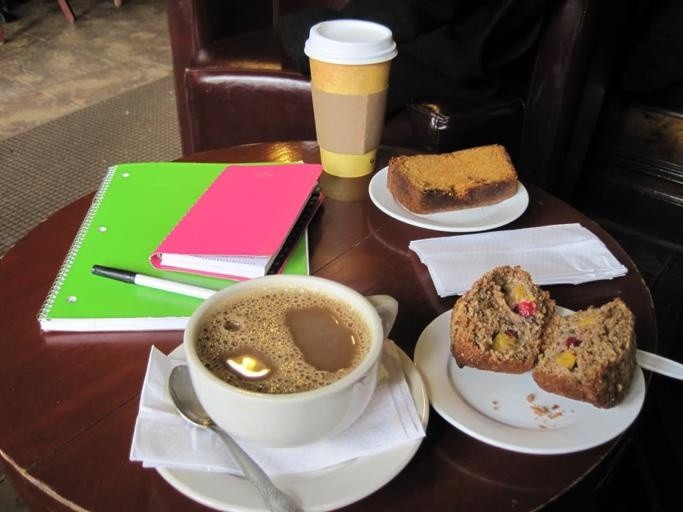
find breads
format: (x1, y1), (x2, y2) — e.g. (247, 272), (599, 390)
(386, 142), (519, 214)
(448, 264), (637, 409)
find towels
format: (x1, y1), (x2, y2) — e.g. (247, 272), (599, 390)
(127, 343), (426, 471)
(409, 221), (629, 300)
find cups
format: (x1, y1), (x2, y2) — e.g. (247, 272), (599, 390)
(184, 273), (400, 449)
(304, 19), (398, 179)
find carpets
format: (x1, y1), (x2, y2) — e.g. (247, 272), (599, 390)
(0, 74), (183, 265)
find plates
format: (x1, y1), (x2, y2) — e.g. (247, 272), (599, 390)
(368, 165), (530, 231)
(155, 337), (427, 512)
(413, 302), (646, 456)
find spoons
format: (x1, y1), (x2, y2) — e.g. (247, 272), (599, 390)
(170, 365), (299, 512)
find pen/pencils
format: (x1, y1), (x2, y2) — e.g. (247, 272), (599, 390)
(92, 265), (220, 300)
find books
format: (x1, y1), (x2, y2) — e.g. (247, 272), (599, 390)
(149, 163), (329, 282)
(38, 160), (311, 333)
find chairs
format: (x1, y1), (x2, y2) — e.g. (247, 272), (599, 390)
(588, 96), (682, 294)
